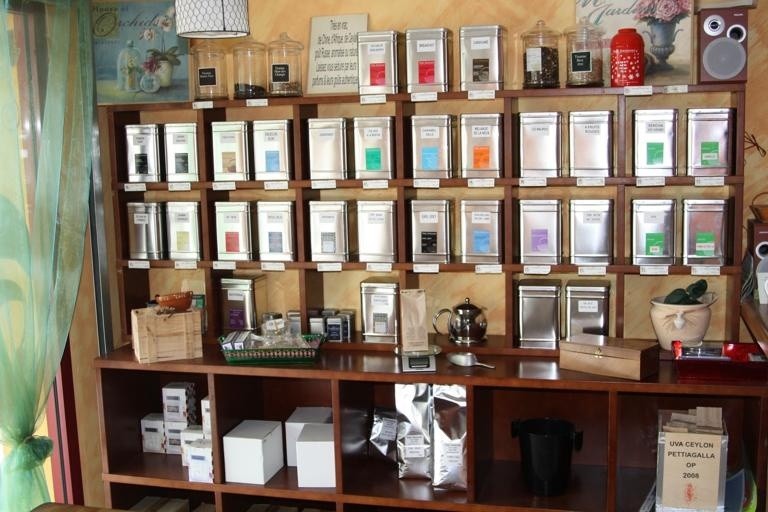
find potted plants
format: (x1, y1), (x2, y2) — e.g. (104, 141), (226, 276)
(649, 279), (718, 350)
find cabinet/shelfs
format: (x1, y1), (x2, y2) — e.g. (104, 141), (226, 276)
(93, 344), (768, 511)
(97, 84), (745, 356)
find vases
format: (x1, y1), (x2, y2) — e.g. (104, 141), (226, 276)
(642, 22), (684, 70)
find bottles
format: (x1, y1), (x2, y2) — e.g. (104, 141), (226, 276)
(140, 55), (162, 94)
(116, 39), (143, 94)
(609, 29), (646, 88)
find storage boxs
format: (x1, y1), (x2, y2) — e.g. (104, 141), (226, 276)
(560, 333), (660, 381)
(130, 307), (203, 363)
(518, 279), (560, 352)
(565, 279), (611, 343)
(359, 276), (399, 343)
(141, 384), (336, 489)
(655, 409), (730, 512)
(219, 274), (269, 336)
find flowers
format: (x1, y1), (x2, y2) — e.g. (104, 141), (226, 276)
(631, 1), (693, 25)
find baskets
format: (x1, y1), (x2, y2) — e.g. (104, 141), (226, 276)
(218, 334), (325, 365)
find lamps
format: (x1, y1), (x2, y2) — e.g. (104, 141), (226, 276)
(175, 0), (251, 39)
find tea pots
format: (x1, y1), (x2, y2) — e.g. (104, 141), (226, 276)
(433, 296), (491, 346)
